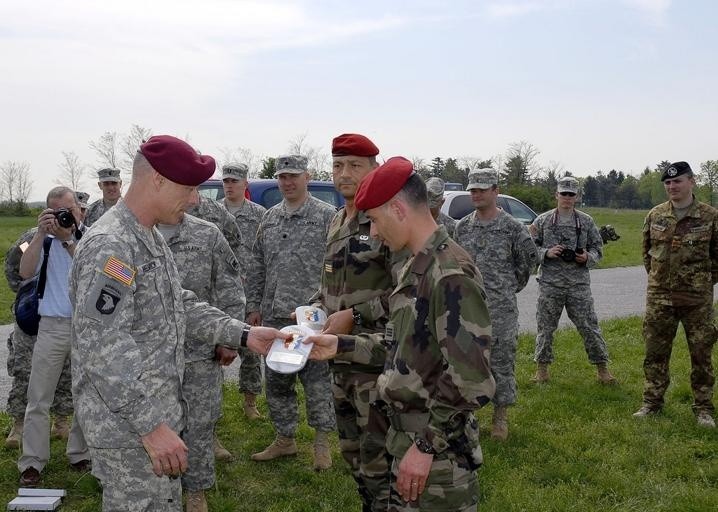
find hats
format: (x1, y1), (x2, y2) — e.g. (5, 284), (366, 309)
(558, 177), (579, 194)
(466, 167), (498, 190)
(75, 192), (91, 209)
(140, 135), (215, 186)
(274, 155), (307, 177)
(354, 156), (413, 210)
(424, 177), (445, 208)
(222, 163), (249, 180)
(98, 168), (121, 183)
(661, 162), (691, 181)
(331, 134), (379, 156)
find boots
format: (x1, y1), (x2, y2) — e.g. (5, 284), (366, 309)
(491, 406), (508, 440)
(7, 418), (23, 449)
(313, 431), (332, 468)
(212, 431), (235, 463)
(250, 433), (297, 461)
(244, 391), (261, 421)
(596, 360), (616, 382)
(530, 362), (548, 382)
(50, 412), (70, 439)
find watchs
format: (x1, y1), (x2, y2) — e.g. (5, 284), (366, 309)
(350, 305), (364, 325)
(414, 438), (436, 455)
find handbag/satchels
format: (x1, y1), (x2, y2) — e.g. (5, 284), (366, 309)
(10, 276), (41, 336)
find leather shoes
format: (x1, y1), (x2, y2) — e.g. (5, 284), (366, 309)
(69, 460), (93, 471)
(18, 467), (41, 488)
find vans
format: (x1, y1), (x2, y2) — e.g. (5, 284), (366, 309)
(195, 179), (345, 210)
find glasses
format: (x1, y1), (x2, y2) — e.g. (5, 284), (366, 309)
(559, 192), (576, 197)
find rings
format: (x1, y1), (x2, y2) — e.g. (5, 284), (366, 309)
(411, 482), (418, 485)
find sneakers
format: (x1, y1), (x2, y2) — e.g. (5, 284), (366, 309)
(634, 406), (663, 418)
(185, 489), (209, 512)
(698, 412), (716, 430)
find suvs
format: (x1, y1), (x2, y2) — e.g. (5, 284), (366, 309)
(440, 191), (540, 230)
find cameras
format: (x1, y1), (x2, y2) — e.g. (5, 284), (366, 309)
(556, 247), (584, 263)
(47, 207), (75, 232)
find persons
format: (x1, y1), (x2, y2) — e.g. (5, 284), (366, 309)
(5, 168), (122, 488)
(185, 163), (266, 461)
(422, 176), (458, 241)
(308, 133), (409, 512)
(150, 212), (245, 512)
(245, 153), (340, 471)
(69, 136), (292, 512)
(524, 176), (613, 385)
(303, 155), (496, 512)
(630, 162), (718, 428)
(453, 168), (538, 439)
